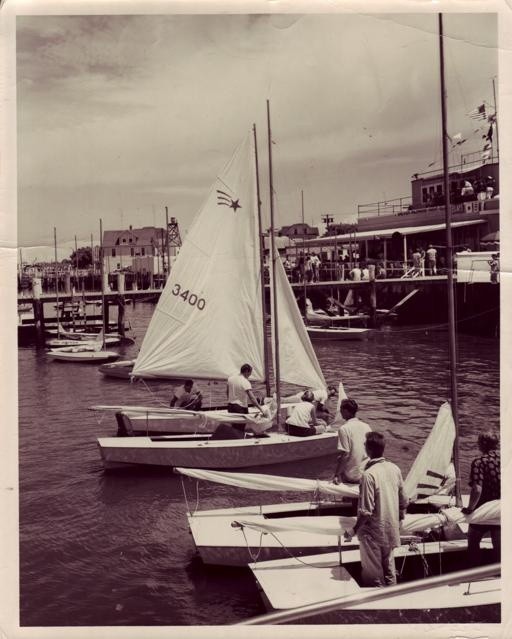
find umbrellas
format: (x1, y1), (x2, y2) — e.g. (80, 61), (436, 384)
(479, 230), (499, 246)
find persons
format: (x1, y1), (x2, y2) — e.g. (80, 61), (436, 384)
(463, 430), (500, 566)
(261, 385), (336, 412)
(227, 364), (266, 438)
(284, 244), (500, 284)
(285, 390), (327, 437)
(334, 398), (374, 516)
(170, 379), (203, 411)
(343, 432), (409, 588)
(461, 175), (495, 200)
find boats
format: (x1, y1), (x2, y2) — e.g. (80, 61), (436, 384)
(306, 301), (400, 327)
(307, 327), (370, 341)
(43, 220), (127, 365)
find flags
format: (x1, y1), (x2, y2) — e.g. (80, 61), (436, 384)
(482, 126), (493, 163)
(465, 103), (488, 121)
